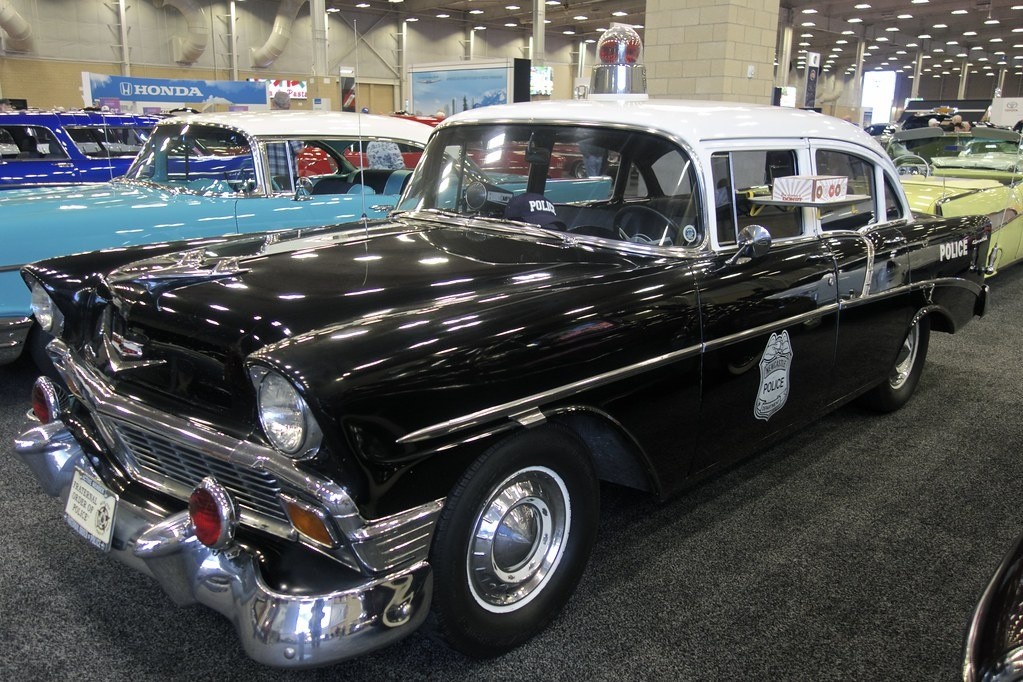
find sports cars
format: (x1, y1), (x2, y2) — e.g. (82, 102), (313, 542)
(887, 126), (1023, 279)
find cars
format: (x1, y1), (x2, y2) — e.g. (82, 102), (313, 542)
(0, 114), (260, 194)
(900, 114), (954, 150)
(0, 112), (501, 393)
(294, 116), (563, 184)
(13, 95), (1015, 670)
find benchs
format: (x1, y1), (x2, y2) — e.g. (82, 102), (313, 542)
(556, 199), (698, 245)
(347, 169), (413, 195)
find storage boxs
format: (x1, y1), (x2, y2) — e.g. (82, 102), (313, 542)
(772, 175), (849, 202)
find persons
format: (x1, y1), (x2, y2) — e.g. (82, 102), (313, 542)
(366, 142), (406, 169)
(928, 118), (940, 128)
(267, 92), (304, 178)
(0, 98), (28, 140)
(952, 115), (971, 133)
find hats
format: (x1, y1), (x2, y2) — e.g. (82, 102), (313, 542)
(362, 108), (369, 114)
(928, 118), (940, 127)
(503, 194), (568, 232)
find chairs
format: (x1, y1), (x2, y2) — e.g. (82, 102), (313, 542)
(18, 136), (40, 158)
(310, 178), (375, 195)
(45, 142), (67, 158)
